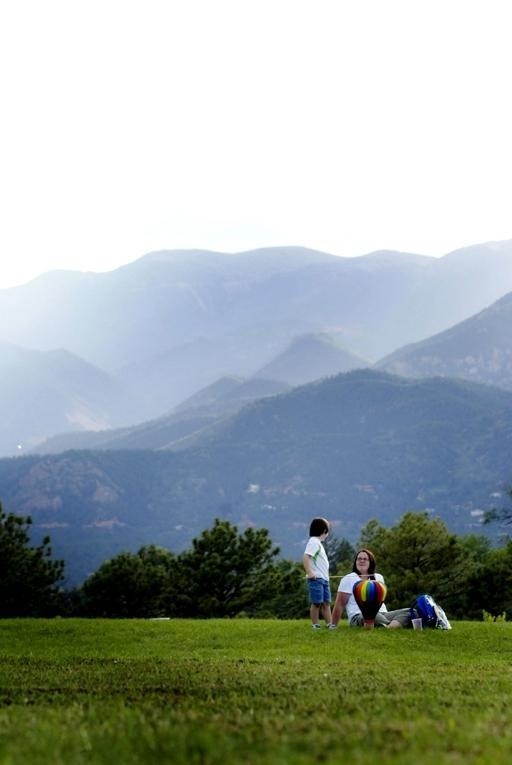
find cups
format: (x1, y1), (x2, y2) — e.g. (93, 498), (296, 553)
(410, 618), (423, 632)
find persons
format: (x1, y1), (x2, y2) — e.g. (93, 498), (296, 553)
(303, 518), (333, 629)
(328, 550), (418, 630)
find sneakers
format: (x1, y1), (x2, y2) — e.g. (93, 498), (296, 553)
(327, 623), (336, 630)
(312, 624), (321, 629)
(374, 622), (388, 628)
